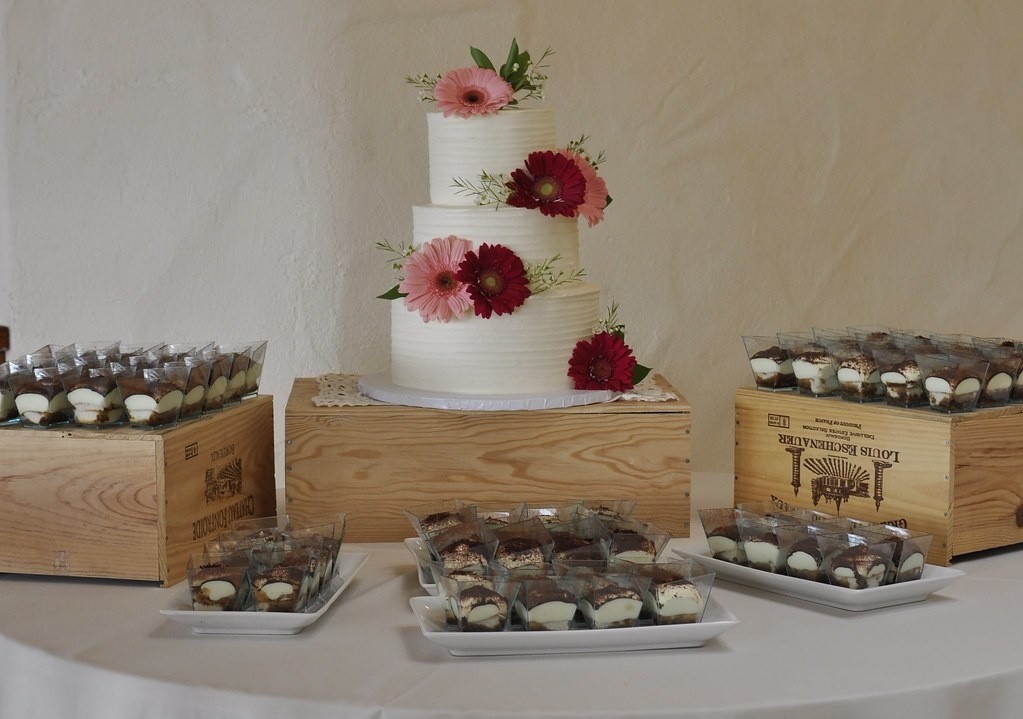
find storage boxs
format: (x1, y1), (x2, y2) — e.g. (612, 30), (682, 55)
(0, 387), (1022, 589)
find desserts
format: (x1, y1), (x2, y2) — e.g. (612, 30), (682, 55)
(742, 326), (1023, 414)
(185, 512), (346, 613)
(697, 500), (934, 591)
(0, 339), (268, 430)
(400, 498), (717, 632)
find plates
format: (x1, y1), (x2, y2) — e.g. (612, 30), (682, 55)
(410, 595), (742, 656)
(672, 547), (965, 612)
(404, 538), (441, 595)
(159, 552), (370, 634)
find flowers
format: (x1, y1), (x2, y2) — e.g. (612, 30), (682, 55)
(370, 237), (593, 326)
(397, 35), (556, 120)
(448, 132), (617, 228)
(567, 302), (657, 394)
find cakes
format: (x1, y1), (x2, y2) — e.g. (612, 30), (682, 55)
(374, 37), (653, 395)
